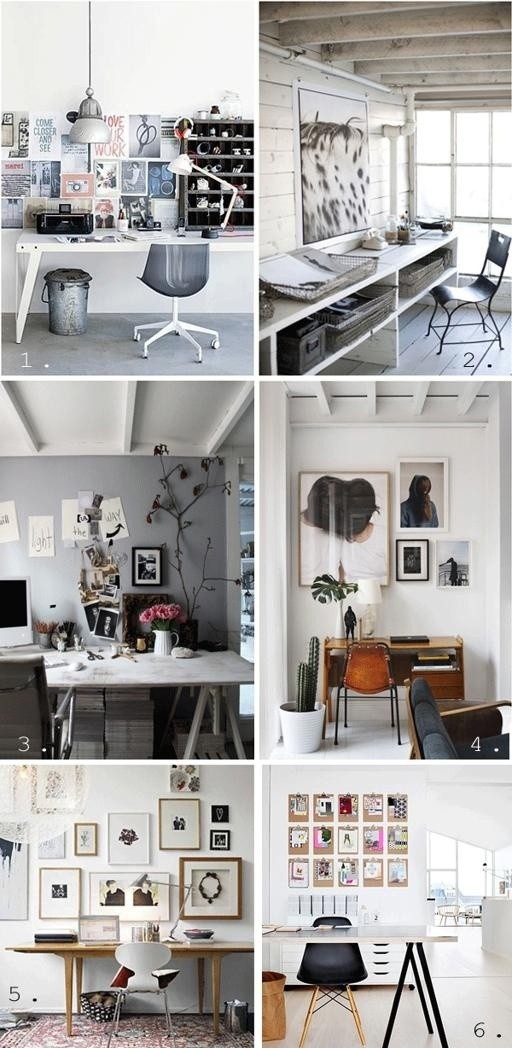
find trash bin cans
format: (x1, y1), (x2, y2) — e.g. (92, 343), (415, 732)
(224, 1001), (248, 1033)
(262, 971), (285, 1041)
(40, 268), (92, 336)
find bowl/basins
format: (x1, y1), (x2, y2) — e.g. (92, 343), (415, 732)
(184, 929), (214, 938)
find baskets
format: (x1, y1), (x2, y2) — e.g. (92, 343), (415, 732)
(314, 283), (399, 354)
(259, 252), (379, 304)
(390, 252), (446, 296)
(79, 990), (124, 1022)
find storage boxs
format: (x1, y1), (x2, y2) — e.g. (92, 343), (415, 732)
(277, 318), (328, 374)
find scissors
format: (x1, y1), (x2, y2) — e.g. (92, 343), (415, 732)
(136, 122), (157, 155)
(87, 650), (103, 660)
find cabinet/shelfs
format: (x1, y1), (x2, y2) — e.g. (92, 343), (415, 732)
(343, 206), (458, 369)
(238, 457), (254, 664)
(178, 116), (254, 231)
(258, 271), (400, 376)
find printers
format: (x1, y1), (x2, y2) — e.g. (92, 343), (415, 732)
(36, 204), (93, 235)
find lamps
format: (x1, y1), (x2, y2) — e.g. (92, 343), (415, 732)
(68, 7), (111, 144)
(355, 578), (382, 640)
(166, 154), (238, 239)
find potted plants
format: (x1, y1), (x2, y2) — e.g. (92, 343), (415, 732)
(279, 636), (326, 755)
(398, 210), (411, 241)
(311, 572), (358, 639)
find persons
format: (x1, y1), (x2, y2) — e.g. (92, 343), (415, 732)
(105, 880), (125, 905)
(344, 606), (357, 640)
(133, 874), (154, 906)
(91, 607), (115, 637)
(449, 558), (458, 586)
(139, 552), (157, 580)
(300, 475), (387, 586)
(401, 474), (439, 527)
(95, 200), (115, 228)
(123, 161), (144, 191)
(174, 816), (186, 829)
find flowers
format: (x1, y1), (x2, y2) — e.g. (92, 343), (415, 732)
(138, 602), (189, 631)
(146, 443), (242, 620)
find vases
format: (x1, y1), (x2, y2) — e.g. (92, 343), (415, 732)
(180, 618), (198, 652)
(151, 629), (179, 657)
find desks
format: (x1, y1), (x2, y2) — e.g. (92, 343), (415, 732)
(0, 643), (254, 759)
(12, 229), (255, 344)
(4, 941), (254, 1037)
(319, 636), (465, 741)
(263, 925), (458, 1048)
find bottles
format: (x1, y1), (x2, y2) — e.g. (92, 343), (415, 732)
(385, 214), (398, 241)
(146, 920), (161, 943)
(210, 106), (221, 120)
(409, 222), (416, 245)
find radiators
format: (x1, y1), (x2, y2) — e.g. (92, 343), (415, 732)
(280, 892), (415, 992)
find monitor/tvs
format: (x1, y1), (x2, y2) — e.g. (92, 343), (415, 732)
(1, 577), (34, 647)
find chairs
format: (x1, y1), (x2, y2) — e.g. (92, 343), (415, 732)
(437, 905), (482, 927)
(133, 244), (220, 363)
(107, 943), (179, 1048)
(425, 230), (511, 355)
(298, 917), (368, 1048)
(334, 642), (402, 746)
(0, 656), (75, 759)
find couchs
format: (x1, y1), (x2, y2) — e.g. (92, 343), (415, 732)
(400, 677), (512, 759)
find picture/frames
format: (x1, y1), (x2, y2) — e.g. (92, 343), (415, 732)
(122, 593), (168, 648)
(132, 546), (163, 587)
(292, 80), (374, 251)
(296, 457), (473, 587)
(93, 158), (121, 198)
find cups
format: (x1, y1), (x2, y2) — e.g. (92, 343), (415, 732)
(135, 637), (148, 653)
(116, 219), (129, 233)
(132, 926), (144, 944)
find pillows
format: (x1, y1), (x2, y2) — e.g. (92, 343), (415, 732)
(109, 965), (178, 988)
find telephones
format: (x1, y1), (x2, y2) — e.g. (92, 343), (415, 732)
(362, 228), (388, 250)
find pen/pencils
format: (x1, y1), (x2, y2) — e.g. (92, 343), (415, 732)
(36, 621), (59, 634)
(119, 207), (128, 218)
(63, 622), (77, 648)
